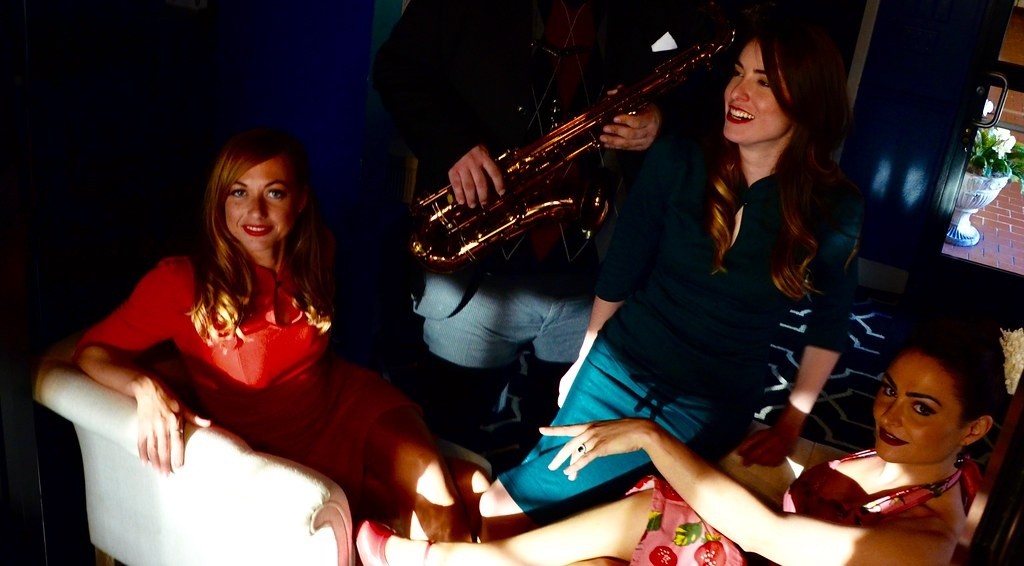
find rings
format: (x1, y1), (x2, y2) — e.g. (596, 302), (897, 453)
(577, 444), (587, 454)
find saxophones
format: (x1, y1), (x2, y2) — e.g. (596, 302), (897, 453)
(404, 1), (737, 273)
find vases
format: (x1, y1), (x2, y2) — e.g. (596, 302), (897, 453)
(945, 166), (1012, 247)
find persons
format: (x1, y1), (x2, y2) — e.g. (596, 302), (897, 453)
(70, 126), (491, 542)
(374, 0), (662, 459)
(478, 24), (859, 542)
(355, 319), (994, 566)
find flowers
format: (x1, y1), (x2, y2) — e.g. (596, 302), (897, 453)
(965, 93), (1024, 195)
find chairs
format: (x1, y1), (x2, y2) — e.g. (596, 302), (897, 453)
(30, 321), (492, 566)
